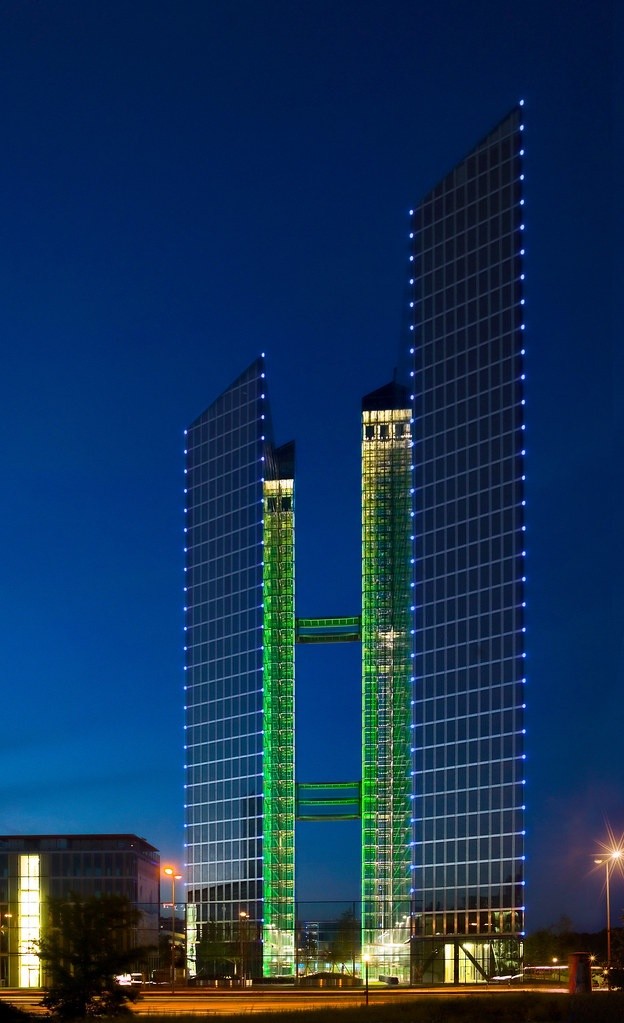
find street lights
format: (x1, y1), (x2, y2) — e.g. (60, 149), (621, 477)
(594, 849), (620, 968)
(164, 867), (183, 986)
(238, 911), (251, 978)
